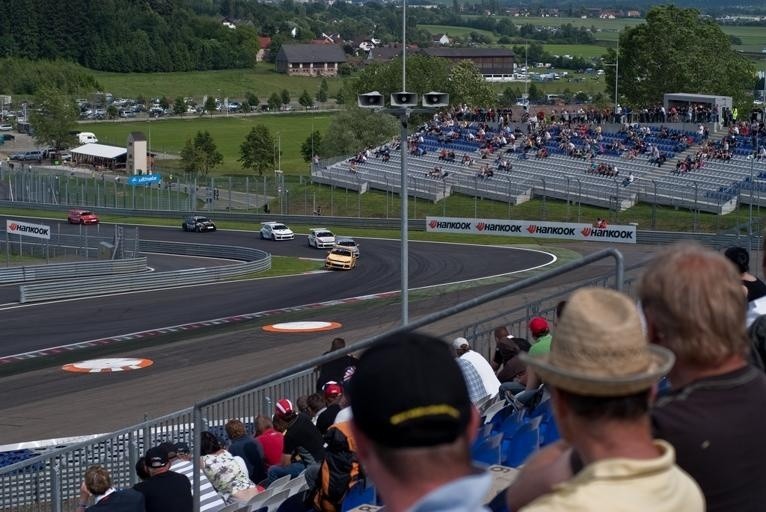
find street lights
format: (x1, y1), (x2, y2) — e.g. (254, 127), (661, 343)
(746, 154), (754, 238)
(517, 37), (528, 99)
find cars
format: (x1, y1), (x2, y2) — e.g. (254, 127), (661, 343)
(285, 106), (296, 111)
(307, 225), (336, 252)
(64, 209), (100, 227)
(336, 237), (360, 259)
(58, 153), (72, 162)
(258, 221), (296, 243)
(183, 212), (217, 233)
(322, 245), (356, 271)
(8, 150), (44, 161)
(0, 92), (242, 131)
(513, 62), (569, 80)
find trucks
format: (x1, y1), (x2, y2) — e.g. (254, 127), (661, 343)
(78, 132), (98, 144)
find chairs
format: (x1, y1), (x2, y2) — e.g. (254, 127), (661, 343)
(217, 324), (673, 512)
(321, 123), (762, 201)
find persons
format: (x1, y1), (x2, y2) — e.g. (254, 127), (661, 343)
(0, 155), (219, 202)
(317, 206), (321, 216)
(347, 101), (765, 183)
(82, 239), (766, 512)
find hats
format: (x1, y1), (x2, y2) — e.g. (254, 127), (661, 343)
(518, 287), (676, 397)
(145, 442), (190, 468)
(528, 318), (550, 334)
(274, 333), (470, 448)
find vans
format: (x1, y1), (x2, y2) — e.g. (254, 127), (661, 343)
(546, 94), (560, 101)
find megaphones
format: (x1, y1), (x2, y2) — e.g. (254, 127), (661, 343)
(391, 91), (418, 109)
(421, 90), (449, 108)
(357, 91), (386, 110)
